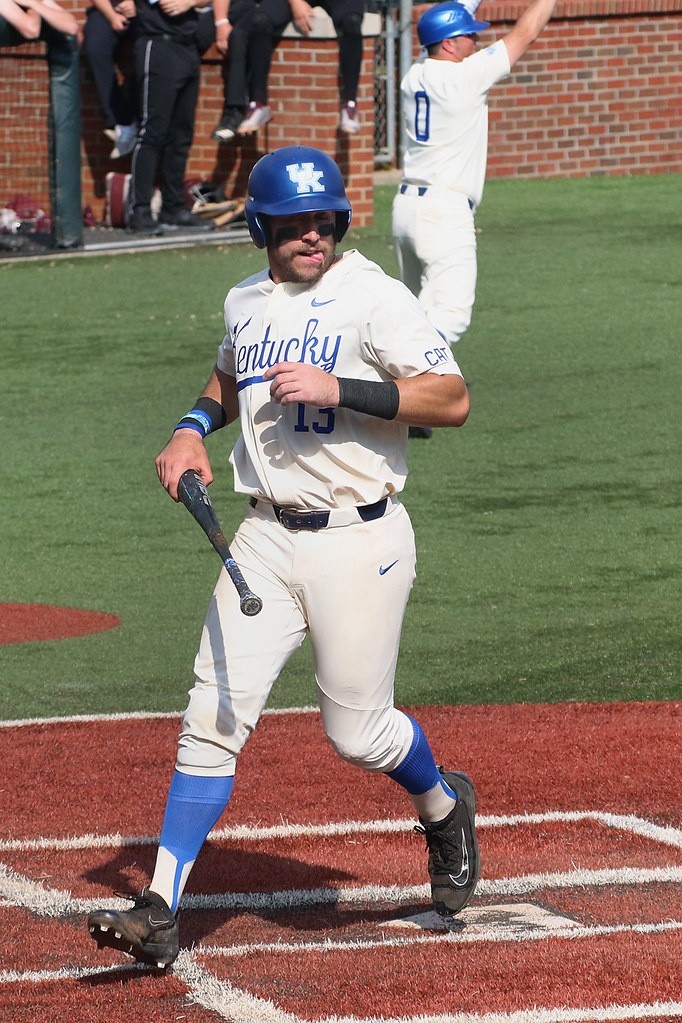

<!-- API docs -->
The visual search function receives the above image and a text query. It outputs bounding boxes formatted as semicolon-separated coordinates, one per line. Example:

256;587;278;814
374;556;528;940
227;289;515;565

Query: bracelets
175;410;212;438
215;18;229;26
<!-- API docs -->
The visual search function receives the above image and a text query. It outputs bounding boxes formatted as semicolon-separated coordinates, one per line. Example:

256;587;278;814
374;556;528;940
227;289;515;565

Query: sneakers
109;119;140;159
210;107;245;143
87;884;181;971
415;765;480;918
236;102;274;137
339;100;360;135
104;129;116;142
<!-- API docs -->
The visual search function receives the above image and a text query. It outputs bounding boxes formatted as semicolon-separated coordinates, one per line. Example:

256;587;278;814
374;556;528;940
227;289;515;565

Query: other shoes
158;209;214;230
127;212;162;235
409;426;432;438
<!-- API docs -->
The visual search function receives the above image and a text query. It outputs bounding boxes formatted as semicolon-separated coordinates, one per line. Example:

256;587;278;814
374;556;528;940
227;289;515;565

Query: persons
391;0;556;347
87;145;481;969
83;0;137;160
196;0;256;143
237;0;364;137
125;0;215;237
0;0;85;251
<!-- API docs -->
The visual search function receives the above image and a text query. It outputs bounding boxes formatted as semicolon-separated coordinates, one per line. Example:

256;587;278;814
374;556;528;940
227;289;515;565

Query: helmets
417;2;491;46
244;146;352;249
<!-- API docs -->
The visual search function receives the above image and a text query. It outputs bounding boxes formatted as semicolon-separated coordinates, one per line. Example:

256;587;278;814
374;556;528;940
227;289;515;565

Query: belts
154;33;190;47
249;496;388;532
400;185;473;210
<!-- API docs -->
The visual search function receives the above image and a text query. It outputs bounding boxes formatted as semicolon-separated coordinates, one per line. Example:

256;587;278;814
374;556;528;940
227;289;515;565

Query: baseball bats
177;468;263;618
